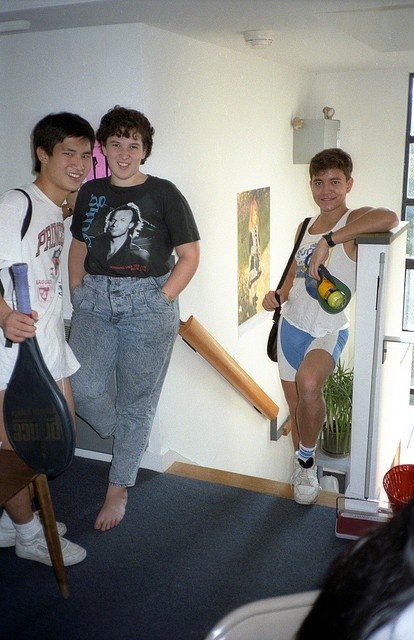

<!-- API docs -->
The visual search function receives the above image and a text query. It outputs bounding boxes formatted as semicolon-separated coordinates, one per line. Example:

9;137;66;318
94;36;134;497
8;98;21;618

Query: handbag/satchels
265;217;311;363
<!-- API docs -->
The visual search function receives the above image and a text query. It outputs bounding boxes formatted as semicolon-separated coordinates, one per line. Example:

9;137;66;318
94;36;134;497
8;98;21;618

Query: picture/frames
237;187;270;325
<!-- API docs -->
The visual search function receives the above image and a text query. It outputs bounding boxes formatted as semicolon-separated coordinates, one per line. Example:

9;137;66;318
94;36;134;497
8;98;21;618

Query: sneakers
15;529;87;568
293;458;320;506
1;509;68;548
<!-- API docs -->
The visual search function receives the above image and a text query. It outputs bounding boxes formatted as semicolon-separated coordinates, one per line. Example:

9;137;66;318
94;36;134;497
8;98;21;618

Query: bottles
316;271;347;310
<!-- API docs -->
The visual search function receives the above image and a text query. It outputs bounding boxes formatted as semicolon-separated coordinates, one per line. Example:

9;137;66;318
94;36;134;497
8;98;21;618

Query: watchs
324;229;336;248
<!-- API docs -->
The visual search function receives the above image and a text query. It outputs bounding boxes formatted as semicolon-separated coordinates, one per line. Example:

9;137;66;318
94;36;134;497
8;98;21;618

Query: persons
290;494;412;640
263;147;399;505
69;106;201;531
0;111;94;568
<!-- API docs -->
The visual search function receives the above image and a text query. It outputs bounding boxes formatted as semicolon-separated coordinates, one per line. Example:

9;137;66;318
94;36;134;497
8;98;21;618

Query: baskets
382;464;414;517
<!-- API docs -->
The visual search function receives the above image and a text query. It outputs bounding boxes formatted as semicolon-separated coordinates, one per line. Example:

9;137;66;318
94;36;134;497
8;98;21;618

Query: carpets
0;457;353;639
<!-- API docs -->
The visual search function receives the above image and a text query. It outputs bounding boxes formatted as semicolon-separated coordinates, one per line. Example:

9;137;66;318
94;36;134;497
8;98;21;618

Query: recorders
335;494;390;540
